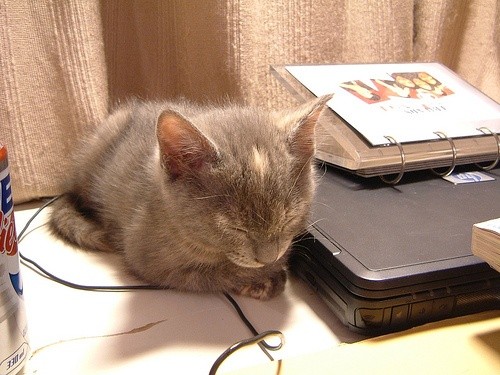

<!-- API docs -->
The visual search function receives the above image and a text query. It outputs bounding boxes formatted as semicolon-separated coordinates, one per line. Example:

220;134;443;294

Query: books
265;63;500;185
471;218;500;273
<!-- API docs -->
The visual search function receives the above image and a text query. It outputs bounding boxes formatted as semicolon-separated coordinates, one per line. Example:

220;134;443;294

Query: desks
10;194;500;375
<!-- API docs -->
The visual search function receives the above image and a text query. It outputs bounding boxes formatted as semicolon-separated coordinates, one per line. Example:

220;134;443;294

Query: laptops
287;160;500;335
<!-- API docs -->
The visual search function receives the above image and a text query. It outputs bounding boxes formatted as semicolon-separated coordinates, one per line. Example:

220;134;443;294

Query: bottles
0;142;32;375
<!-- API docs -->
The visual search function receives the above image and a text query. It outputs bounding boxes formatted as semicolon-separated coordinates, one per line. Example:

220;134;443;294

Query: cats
46;87;334;301
